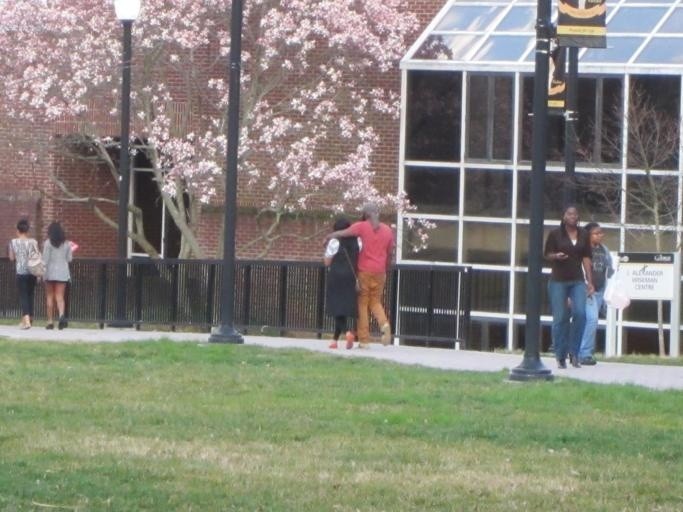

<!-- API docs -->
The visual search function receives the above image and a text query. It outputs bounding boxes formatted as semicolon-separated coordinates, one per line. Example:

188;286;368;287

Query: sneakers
328;324;391;350
17;316;69;330
555;356;596;368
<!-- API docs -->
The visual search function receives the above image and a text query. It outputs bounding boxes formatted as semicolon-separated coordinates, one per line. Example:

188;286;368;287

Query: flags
556;0;608;50
547;25;568;119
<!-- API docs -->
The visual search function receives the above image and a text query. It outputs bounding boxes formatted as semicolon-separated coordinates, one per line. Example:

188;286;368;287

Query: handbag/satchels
355;277;362;293
603;273;630;309
27;245;46;278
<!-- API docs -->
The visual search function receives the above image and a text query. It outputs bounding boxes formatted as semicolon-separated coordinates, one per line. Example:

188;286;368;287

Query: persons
38;220;73;331
540;203;595;370
577;221;614;367
320;216;363;351
319;204;396;352
7;218;40;331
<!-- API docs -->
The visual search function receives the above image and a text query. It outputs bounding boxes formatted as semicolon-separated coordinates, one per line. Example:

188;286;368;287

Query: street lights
111;1;141;327
